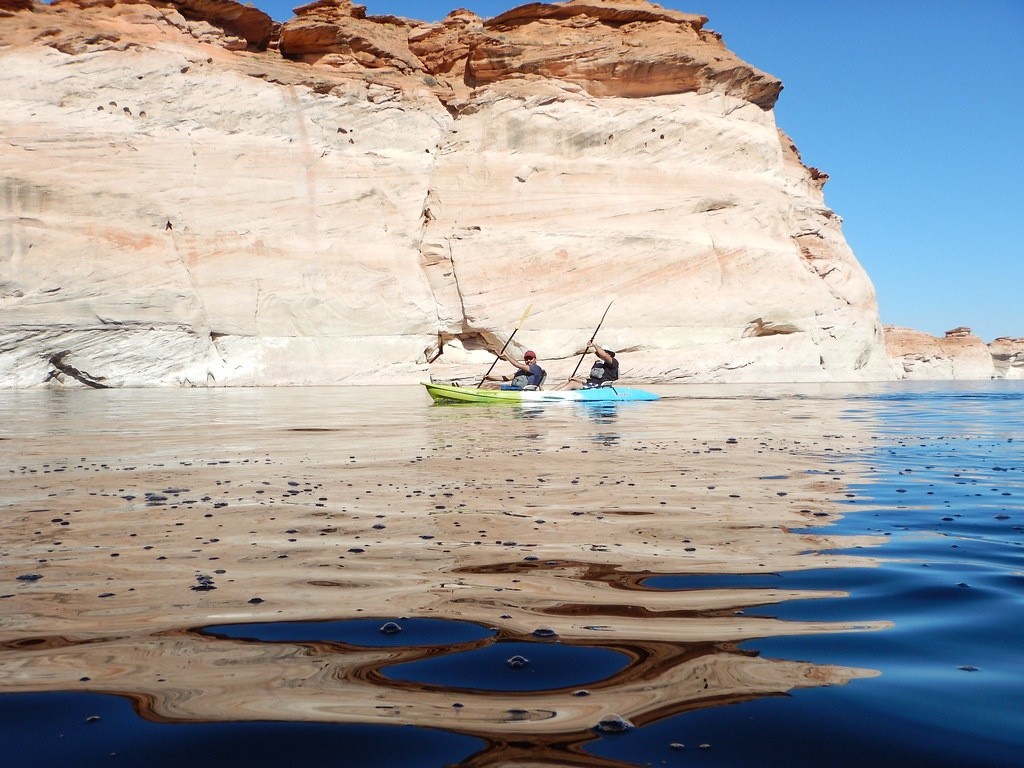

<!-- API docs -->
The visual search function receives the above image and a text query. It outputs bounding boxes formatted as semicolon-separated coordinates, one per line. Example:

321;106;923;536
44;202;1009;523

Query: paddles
476;304;533;390
550;300;614;390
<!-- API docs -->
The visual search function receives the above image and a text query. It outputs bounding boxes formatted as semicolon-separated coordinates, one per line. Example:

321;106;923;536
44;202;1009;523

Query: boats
420;381;664;405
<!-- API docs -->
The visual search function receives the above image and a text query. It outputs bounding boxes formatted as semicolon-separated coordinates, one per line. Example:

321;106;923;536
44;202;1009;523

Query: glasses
525;357;536;361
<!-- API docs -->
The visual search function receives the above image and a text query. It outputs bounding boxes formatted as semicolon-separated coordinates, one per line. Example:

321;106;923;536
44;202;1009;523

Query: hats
595;345;615;358
524;350;536;359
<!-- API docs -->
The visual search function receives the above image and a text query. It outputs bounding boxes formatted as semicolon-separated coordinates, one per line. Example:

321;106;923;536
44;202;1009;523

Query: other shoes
456;382;459;387
452;382;455;387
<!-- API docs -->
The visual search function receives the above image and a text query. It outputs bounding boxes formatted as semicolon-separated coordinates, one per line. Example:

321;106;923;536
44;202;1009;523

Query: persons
550;340;619;391
452;351;542;391
